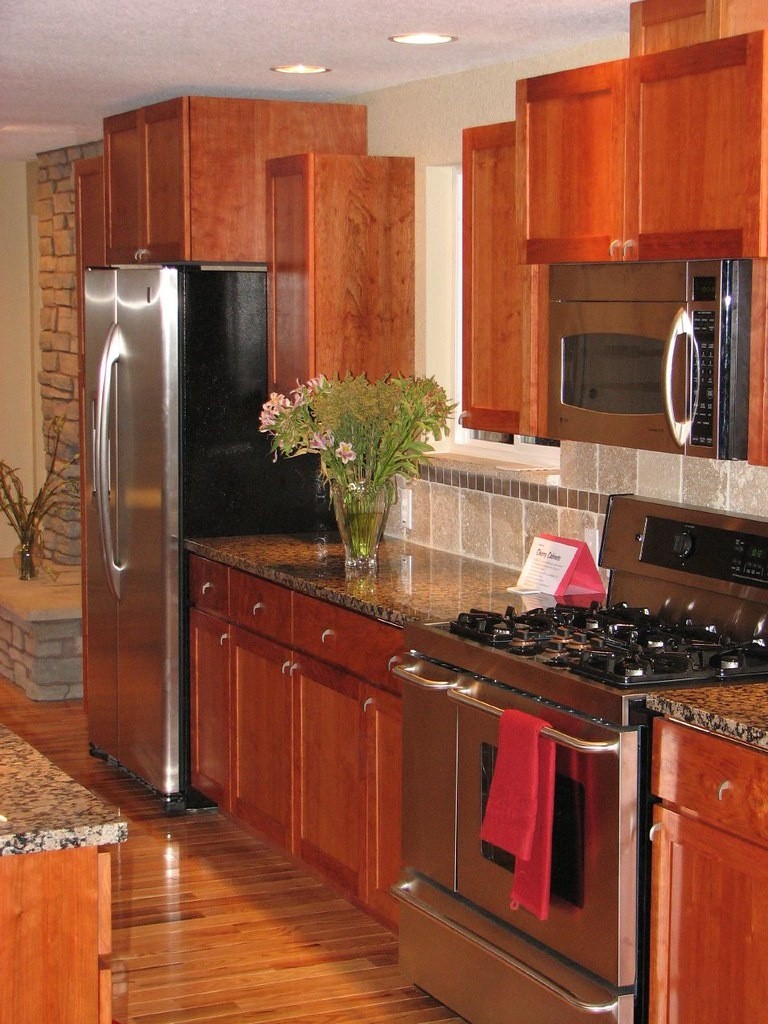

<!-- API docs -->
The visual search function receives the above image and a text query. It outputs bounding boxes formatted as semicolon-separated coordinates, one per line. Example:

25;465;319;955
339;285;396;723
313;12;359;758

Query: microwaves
545;256;754;462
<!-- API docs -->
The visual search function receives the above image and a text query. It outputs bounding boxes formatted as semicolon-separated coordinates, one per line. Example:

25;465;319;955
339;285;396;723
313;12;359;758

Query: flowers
259;372;458;561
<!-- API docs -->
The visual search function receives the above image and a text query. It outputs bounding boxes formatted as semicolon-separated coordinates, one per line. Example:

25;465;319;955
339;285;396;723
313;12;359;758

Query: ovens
386;655;651;1024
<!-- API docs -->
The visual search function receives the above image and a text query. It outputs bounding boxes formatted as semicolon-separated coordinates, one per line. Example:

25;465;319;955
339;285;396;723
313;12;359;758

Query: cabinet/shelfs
515;1;768;264
462;120;526;434
188;552;404;936
103;96;368;263
266;152;415;403
0;844;114;1024
649;714;768;1024
74;155;105;713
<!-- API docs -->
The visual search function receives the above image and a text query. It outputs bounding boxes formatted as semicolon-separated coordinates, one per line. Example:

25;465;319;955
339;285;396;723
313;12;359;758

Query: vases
329;481;394;569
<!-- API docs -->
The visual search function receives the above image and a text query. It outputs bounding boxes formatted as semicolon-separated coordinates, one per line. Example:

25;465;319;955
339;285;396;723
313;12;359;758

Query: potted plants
1;402;79;581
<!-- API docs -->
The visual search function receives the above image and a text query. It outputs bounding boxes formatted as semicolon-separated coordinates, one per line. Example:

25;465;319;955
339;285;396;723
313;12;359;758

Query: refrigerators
80;261;270;817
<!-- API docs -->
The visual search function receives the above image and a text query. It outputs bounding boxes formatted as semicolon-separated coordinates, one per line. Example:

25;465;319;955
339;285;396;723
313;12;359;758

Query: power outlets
399;554;411;596
401;489;412;528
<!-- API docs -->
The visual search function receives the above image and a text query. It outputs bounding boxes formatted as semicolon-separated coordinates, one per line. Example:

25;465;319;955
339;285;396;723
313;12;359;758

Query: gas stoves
401;598;768;691
